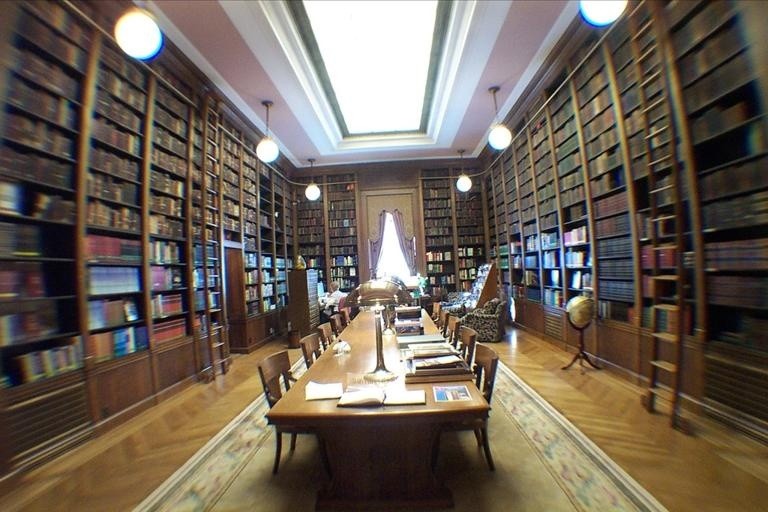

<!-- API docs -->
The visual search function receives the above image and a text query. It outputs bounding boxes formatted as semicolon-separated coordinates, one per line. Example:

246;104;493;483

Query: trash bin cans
289;330;301;348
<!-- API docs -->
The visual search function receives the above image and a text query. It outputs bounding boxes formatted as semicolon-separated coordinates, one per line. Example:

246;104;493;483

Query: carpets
126;329;671;512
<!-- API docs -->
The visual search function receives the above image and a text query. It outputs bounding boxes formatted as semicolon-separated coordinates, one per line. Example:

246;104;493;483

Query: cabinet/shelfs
288;269;323;339
469;262;498;310
566;1;699;401
478;43;596;365
80;2;226;435
294;158;486;299
647;0;768;436
219;91;296;365
2;1;104;497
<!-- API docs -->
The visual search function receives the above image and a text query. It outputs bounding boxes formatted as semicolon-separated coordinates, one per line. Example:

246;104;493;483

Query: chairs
255;302;499;511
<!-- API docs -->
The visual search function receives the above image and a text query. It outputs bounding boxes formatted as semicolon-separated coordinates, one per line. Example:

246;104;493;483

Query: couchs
437;291;508;343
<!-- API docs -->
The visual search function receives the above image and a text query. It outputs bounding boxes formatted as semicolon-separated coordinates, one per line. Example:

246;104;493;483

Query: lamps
487;85;513;150
305;157;320;200
456;148;472;193
255;100;279;164
346;279;415;382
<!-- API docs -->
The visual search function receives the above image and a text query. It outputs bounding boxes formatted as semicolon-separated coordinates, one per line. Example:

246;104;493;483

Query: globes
562;296;603;371
